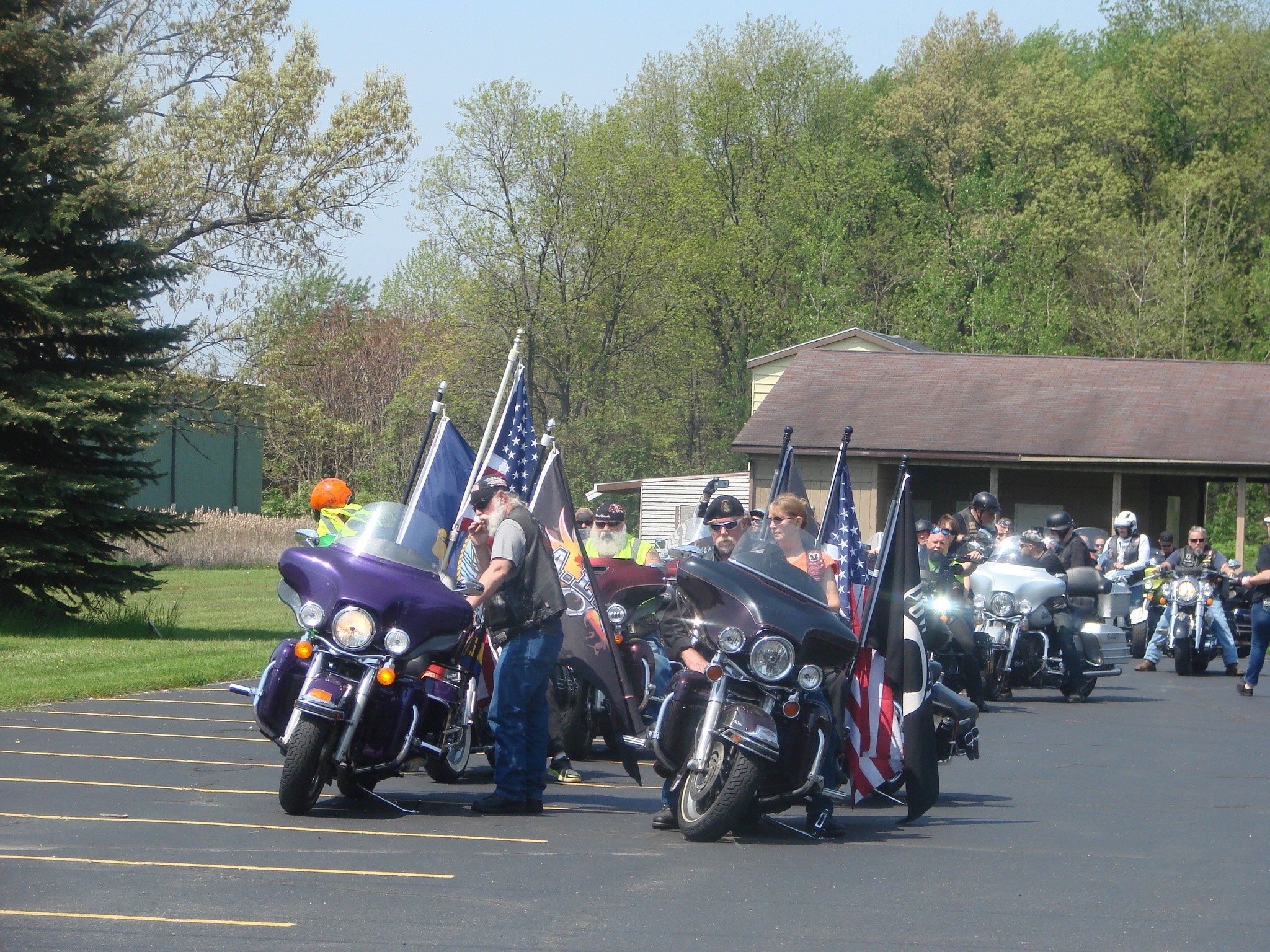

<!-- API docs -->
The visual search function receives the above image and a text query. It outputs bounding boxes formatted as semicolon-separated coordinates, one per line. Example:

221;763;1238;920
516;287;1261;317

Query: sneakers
547;755;582;783
652;803;679;828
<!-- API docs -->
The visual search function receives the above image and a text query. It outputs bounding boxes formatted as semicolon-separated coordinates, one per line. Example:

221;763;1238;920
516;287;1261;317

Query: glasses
472;494;494;512
1162;545;1172;548
576;521;594;526
996;533;1004;537
1018;544;1030;548
931;527;949;535
1189;538;1205;543
595;521;620;528
764;517;795;526
944;528;959;536
708;516;747;532
1093;544;1105;547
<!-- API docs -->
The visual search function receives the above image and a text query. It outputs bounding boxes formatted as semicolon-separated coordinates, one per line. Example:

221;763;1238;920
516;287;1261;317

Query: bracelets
1249;578;1254;586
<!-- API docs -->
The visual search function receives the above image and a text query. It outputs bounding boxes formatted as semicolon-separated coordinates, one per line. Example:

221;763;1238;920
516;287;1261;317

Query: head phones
1133;528;1140;538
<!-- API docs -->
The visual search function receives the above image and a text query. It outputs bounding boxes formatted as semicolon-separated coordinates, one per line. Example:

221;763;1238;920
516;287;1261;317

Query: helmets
1114;510;1137;535
750;508;766;520
915;519;934;531
310;478;356;512
972;492;1001;512
1045;511;1076;530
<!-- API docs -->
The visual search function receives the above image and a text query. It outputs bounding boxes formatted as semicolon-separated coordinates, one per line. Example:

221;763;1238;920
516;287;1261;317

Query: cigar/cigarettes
475;520;486;533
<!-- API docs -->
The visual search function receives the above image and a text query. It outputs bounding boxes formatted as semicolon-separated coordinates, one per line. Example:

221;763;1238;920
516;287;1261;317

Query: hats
595;502;625;521
574;508;594;521
997;517;1013;528
1159;531;1174;543
1021;529;1043;542
703;496;744;525
470;474;510;504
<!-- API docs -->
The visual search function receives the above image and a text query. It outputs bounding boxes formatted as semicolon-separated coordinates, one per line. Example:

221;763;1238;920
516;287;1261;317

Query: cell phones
714;480;729;488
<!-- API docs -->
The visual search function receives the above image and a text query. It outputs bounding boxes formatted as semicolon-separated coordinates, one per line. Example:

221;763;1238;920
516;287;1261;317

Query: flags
759;443;939;828
397;368;646;786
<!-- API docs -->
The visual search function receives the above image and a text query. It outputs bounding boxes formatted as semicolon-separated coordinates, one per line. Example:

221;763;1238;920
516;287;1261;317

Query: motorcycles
863;527;1256;806
547;515;716;759
230;500;496;816
622;520;851;843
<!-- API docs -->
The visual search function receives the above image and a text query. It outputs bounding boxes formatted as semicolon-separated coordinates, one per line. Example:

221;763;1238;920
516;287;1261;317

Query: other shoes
995;689;1013;701
807;812;845;836
968;698;989;712
1134;659;1156;671
1066;687;1082;703
1236;678;1253;696
471;792;544;814
1224;664;1246;676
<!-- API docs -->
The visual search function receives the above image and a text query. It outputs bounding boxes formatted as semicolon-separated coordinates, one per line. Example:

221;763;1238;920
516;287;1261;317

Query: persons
913;493;1106;702
574;501;674;738
547;689;581;782
1236;510;1270;696
651;479;849;837
1133;526;1246;676
1102;511;1150;646
461;474;580;816
1149;531;1177;565
